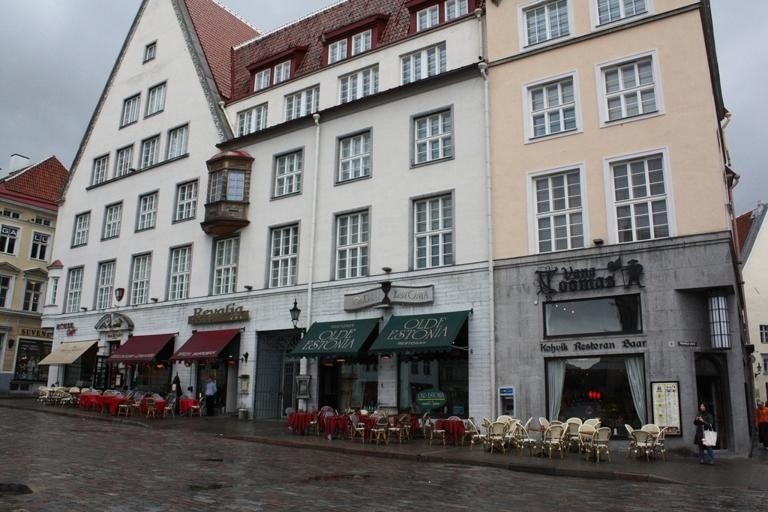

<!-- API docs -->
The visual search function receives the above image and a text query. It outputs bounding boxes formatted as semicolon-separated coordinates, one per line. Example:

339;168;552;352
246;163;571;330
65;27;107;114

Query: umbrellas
172;372;182;414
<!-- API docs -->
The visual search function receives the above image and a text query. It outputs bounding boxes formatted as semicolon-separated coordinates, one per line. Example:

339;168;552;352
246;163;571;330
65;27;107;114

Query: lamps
114;287;124;302
707;296;732;349
290;298;305;332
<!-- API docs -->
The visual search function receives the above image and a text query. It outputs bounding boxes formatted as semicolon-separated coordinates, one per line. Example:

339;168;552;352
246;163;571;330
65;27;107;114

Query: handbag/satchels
702;430;718;447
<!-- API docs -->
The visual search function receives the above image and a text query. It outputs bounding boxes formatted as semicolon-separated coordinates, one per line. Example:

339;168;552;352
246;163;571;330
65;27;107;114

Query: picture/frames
650;380;683;438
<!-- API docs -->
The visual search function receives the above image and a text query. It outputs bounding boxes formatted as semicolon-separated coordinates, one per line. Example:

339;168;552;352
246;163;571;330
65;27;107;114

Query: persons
753;400;768;450
182;387;199;416
203;375;218;416
692;401;715;466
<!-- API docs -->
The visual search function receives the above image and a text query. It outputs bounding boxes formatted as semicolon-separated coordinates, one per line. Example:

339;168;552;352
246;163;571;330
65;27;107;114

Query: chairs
36;385;206;419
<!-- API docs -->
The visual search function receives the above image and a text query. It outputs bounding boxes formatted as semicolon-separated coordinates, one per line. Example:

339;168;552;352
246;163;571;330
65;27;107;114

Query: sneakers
700;460;704;464
709;460;714;465
